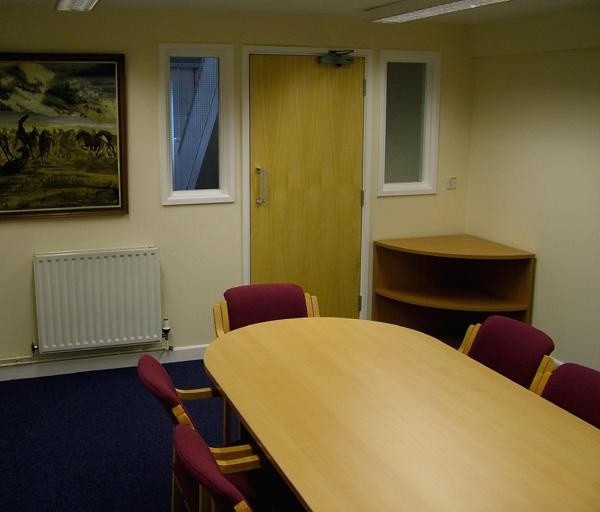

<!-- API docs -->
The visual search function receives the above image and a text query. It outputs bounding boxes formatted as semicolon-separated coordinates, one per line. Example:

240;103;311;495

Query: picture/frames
0;52;129;220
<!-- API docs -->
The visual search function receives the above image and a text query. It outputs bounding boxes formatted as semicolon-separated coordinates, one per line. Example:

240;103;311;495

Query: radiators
33;246;162;354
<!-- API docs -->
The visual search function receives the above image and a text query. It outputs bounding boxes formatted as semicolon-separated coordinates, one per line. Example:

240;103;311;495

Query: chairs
212;282;321;446
138;353;257;512
535;358;600;429
458;313;555;391
173;423;270;512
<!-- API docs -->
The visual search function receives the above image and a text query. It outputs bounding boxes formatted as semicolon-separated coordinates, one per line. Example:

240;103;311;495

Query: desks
203;315;600;512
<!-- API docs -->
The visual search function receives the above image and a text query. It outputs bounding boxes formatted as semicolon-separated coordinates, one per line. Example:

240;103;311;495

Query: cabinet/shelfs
370;236;536;349
